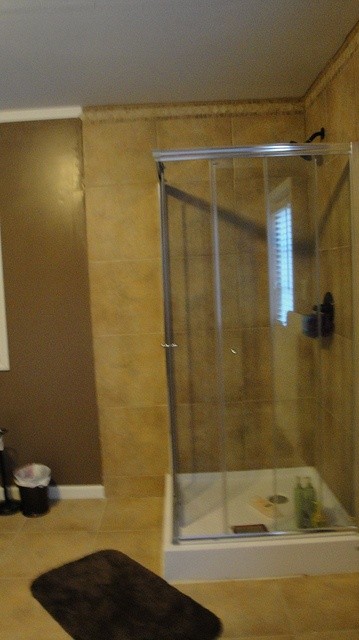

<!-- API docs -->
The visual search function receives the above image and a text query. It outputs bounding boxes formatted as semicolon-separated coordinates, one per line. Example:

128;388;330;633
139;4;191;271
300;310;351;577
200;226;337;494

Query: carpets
30;550;222;640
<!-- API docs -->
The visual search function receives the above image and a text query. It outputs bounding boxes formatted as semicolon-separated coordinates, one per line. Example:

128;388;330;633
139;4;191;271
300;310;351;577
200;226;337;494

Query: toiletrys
294;475;306;529
306;475;318;528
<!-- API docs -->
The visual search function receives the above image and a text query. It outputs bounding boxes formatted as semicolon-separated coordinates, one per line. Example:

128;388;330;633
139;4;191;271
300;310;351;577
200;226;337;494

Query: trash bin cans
13;464;52;518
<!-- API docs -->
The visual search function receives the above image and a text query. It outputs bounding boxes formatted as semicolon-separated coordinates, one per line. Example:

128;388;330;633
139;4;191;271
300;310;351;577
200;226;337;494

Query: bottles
306;477;317;531
1;427;18;518
293;478;304;531
